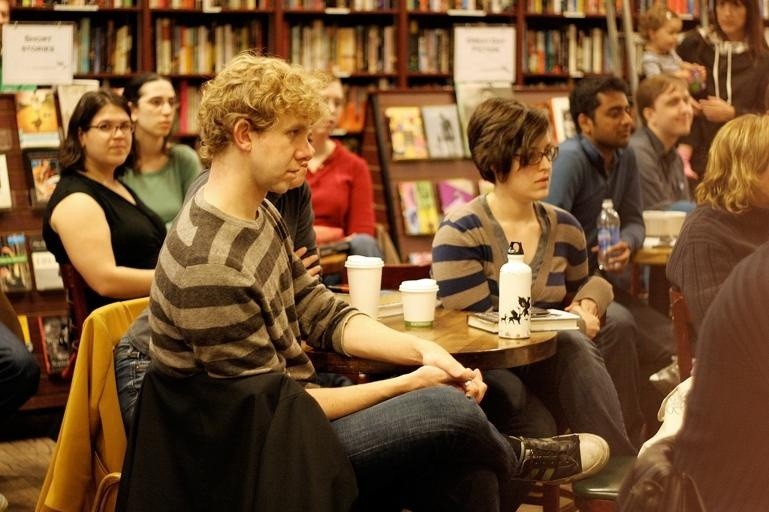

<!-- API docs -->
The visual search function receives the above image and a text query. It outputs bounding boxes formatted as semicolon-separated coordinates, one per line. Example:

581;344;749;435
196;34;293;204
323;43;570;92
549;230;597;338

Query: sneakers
649;356;696;397
508;432;610;484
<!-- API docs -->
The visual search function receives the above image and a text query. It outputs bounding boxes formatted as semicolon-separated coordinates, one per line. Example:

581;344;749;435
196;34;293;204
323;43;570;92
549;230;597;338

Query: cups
399;278;440;329
344;254;385;320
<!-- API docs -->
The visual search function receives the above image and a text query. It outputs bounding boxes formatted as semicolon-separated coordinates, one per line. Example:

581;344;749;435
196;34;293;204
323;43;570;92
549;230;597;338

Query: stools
574;453;637;512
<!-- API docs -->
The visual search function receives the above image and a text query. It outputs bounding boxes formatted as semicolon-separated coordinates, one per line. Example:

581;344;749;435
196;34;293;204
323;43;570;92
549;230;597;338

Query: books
523;0;715;77
385;103;494;266
0;0;142;377
551;97;576;143
466;306;582;334
149;0;516;137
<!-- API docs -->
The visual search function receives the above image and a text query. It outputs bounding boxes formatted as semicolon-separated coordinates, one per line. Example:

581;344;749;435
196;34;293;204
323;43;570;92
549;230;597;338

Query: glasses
515;146;558;165
90;122;134;133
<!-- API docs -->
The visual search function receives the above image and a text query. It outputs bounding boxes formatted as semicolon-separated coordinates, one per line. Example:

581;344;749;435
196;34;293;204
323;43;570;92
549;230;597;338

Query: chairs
33;268;573;512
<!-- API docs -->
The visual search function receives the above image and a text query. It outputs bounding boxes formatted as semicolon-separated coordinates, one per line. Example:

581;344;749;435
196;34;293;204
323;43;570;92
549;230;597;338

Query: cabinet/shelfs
0;0;769;412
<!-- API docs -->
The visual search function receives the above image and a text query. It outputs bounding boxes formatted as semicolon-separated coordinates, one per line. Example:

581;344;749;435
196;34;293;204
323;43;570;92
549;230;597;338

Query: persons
0;321;40;423
431;96;638;457
670;239;769;512
121;71;205;228
41;89;168;316
147;54;611;512
292;70;375;255
536;73;674;436
114;132;323;434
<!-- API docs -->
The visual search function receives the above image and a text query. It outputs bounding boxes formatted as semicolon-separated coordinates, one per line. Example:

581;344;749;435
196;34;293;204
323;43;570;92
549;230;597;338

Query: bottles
497;241;533;340
597;198;620;270
